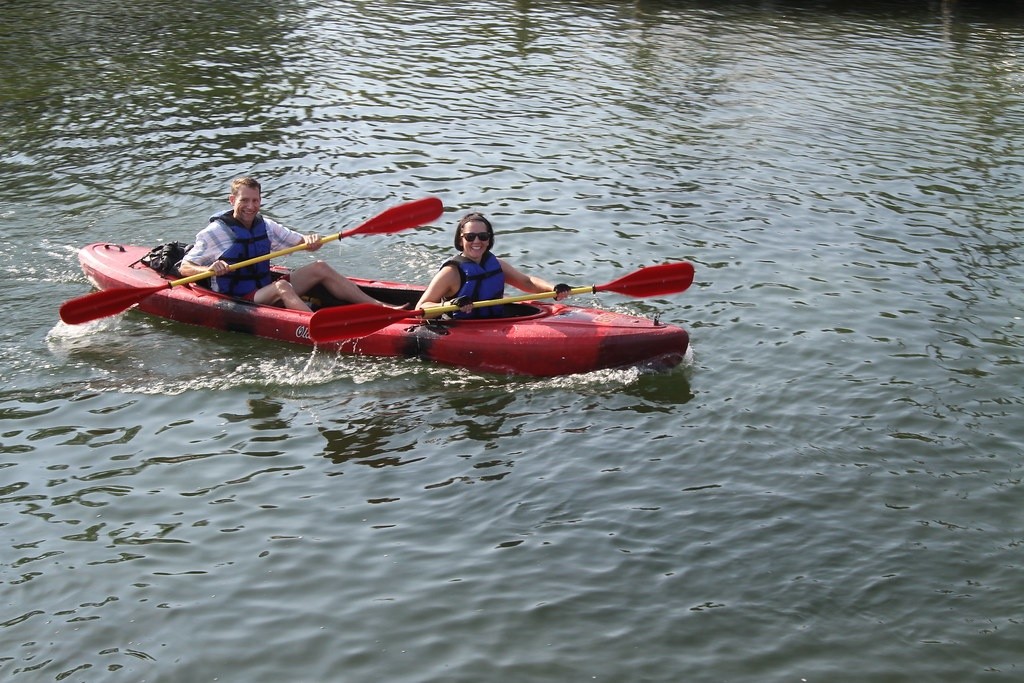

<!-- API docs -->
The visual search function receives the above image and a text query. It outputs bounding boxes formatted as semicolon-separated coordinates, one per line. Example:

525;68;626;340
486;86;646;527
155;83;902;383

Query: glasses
460;232;491;243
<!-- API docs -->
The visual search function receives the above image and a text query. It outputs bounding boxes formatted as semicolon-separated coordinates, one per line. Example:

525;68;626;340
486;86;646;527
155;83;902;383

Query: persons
414;212;572;319
178;177;411;311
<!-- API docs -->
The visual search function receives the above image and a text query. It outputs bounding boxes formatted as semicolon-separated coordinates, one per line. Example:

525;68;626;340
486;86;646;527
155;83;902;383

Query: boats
77;239;689;381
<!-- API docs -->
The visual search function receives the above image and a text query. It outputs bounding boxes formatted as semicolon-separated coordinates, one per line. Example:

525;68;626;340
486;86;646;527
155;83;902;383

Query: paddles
307;263;694;348
58;196;446;327
